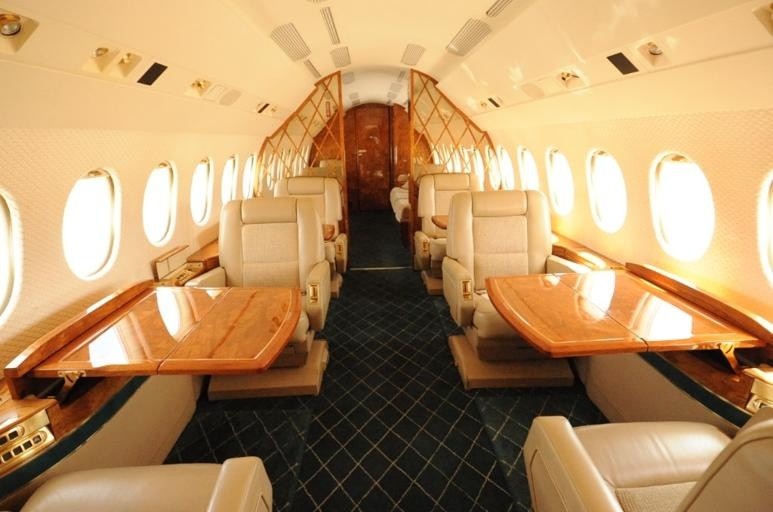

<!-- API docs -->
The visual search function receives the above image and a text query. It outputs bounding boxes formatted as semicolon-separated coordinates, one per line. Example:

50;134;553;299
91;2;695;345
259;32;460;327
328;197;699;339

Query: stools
19;457;273;512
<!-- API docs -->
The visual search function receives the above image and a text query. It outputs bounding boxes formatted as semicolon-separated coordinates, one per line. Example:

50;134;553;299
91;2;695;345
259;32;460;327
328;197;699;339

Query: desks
485;267;767;375
26;285;302;403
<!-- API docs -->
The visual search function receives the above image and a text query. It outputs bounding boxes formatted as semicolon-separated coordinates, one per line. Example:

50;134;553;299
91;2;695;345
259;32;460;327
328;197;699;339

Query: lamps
648;42;663;55
0;13;21;36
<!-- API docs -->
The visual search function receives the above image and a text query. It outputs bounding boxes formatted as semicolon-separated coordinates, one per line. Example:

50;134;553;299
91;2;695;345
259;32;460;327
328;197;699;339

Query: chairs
522;406;773;512
389;163;447;235
184;159;347;402
412;172;479;289
441;188;591;361
